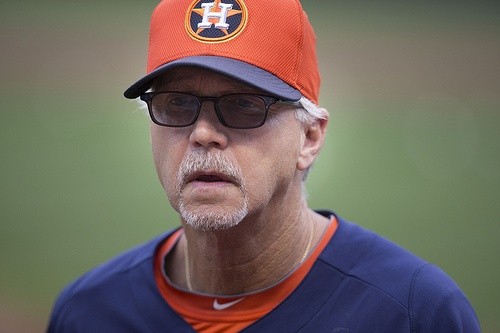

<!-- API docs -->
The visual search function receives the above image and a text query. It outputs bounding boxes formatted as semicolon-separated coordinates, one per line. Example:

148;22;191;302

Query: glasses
139;90;280;129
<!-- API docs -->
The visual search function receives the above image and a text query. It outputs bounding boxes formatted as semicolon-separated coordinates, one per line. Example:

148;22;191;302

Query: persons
46;0;482;332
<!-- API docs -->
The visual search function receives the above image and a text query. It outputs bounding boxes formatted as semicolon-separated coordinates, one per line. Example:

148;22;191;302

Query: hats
123;0;321;106
